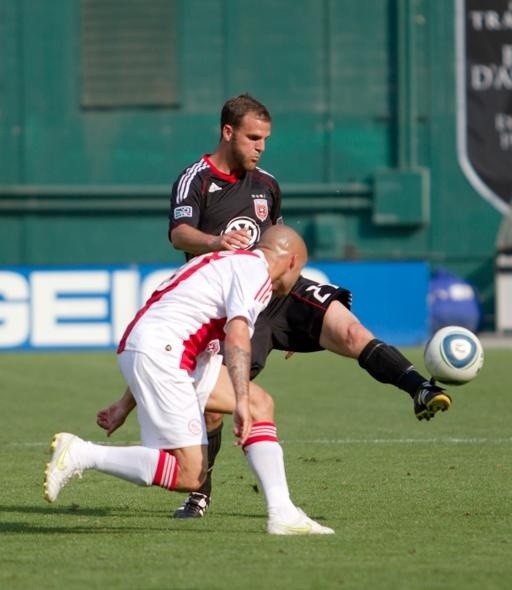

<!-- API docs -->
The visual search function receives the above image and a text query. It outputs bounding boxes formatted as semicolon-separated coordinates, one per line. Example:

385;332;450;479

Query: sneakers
265;509;334;537
43;432;81;502
173;491;211;519
414;380;452;422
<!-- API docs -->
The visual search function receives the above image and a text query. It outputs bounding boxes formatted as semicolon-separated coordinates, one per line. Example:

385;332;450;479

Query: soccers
423;325;484;386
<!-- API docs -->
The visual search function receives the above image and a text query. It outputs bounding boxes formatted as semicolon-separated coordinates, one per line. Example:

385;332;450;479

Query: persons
42;224;336;537
168;93;453;521
